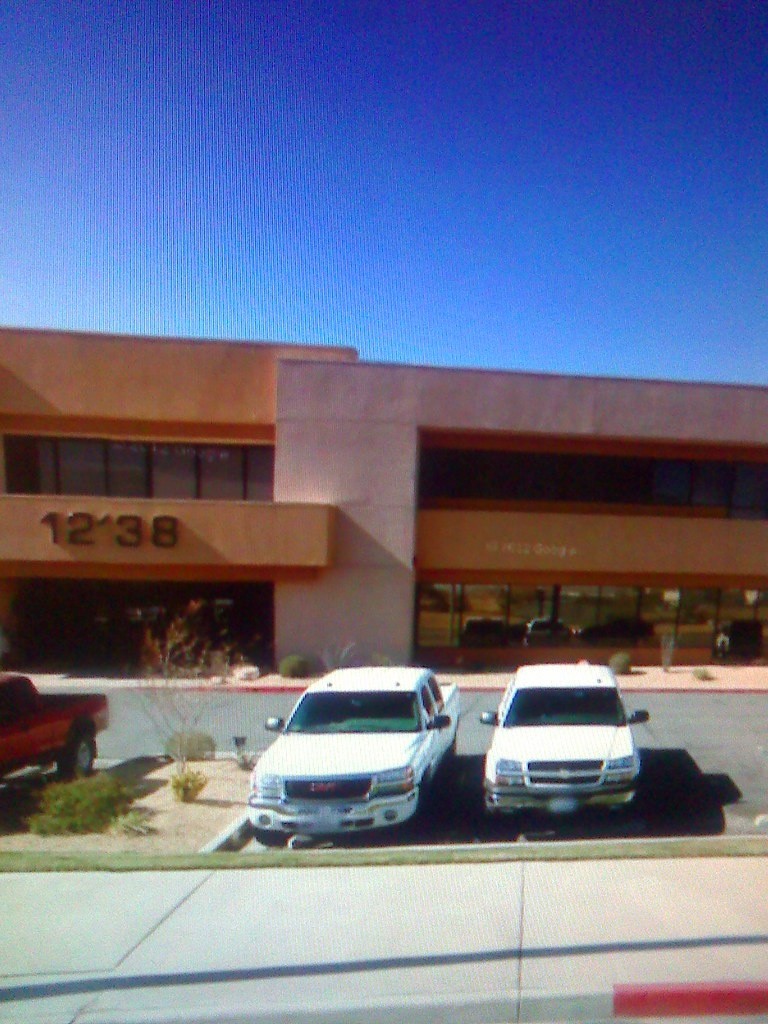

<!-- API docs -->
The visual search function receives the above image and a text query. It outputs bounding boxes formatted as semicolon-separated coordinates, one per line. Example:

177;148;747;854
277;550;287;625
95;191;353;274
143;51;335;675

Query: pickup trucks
0;673;108;783
245;664;462;845
481;661;651;815
